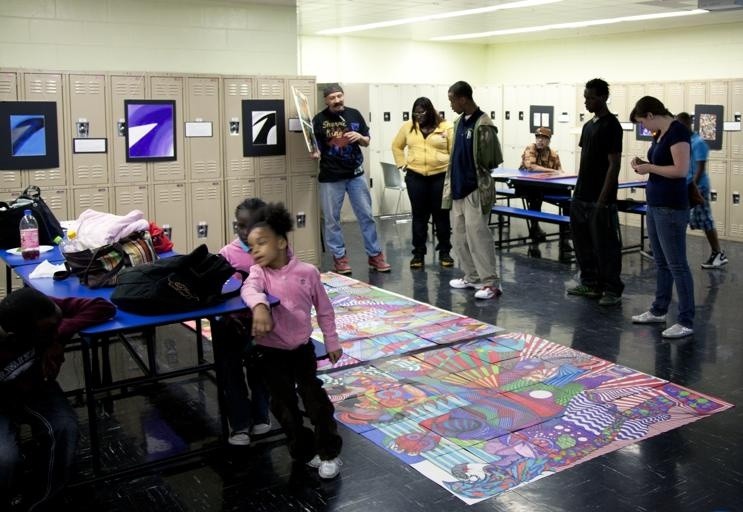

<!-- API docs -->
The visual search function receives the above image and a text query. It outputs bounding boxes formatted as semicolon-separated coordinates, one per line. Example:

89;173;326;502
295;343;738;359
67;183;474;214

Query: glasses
413;110;428;117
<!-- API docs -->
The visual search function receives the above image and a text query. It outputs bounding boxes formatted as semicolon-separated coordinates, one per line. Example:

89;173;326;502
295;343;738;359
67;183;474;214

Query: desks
0;223;67;302
6;249;296;500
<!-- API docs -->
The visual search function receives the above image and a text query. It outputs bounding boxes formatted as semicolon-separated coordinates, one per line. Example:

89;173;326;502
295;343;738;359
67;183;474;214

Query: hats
322;83;343;97
535;127;551;138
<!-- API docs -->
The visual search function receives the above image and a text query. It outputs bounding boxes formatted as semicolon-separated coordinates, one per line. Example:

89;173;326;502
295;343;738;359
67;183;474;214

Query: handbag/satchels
0;185;66;252
52;208;174;288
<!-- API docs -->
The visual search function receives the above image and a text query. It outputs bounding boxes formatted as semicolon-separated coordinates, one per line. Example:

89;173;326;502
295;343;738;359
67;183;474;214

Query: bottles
52;236;80;258
64;229;94;255
18;208;39;261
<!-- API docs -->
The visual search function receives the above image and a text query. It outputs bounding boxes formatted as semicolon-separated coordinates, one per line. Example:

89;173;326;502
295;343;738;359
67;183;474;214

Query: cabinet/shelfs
315;76;742;245
0;67;321;338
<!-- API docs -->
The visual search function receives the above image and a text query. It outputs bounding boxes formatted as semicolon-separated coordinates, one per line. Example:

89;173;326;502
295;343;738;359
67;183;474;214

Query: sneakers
473;282;503;300
566;284;600;297
640;248;656;261
333;249;354;275
631;310;667;324
409;255;424;268
439;255;455;267
661;322;694;338
227;427;250;446
449;277;483;289
599;291;622;306
252;414;273;436
530;227;546;239
306;453;322;468
318;453;343;479
367;252;393;272
701;249;729;268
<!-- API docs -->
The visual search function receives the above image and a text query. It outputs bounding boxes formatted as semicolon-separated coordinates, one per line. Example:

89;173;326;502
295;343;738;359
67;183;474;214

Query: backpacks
108;244;248;312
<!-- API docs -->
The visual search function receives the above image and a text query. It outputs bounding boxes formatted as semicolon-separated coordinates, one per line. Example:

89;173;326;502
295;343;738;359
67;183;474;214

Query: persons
0;288;117;512
241;201;343;479
567;78;626;305
213;198;293;445
640;112;728;268
441;81;504;299
391;96;455;266
629;96;695;339
311;84;391;274
514;127;574;252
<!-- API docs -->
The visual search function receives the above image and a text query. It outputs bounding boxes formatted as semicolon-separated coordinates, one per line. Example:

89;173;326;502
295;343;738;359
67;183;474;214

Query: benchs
308;335;331;361
427;163;657;277
